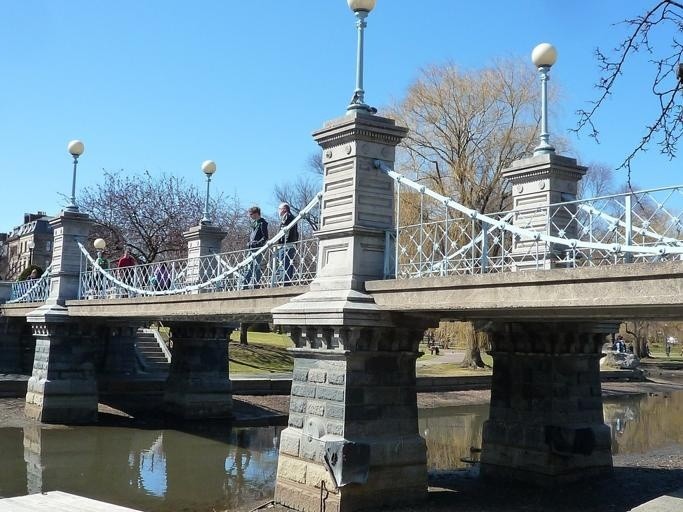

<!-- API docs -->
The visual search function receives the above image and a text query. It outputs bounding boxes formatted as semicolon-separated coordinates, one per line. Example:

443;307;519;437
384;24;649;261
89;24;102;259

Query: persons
93;251;108;290
428;347;433;355
23;269;38;302
118;251;134;297
277;203;297;286
435;347;438;354
242;207;267;290
616;335;683;359
149;261;169;291
615;416;626;436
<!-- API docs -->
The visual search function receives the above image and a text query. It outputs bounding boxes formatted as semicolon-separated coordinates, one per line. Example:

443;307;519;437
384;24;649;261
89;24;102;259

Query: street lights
64;140;84;213
27;241;35;266
664;323;667;355
198;159;215;227
345;0;379;118
271;425;277;450
423;408;430;436
531;43;557;158
91;238;106;277
